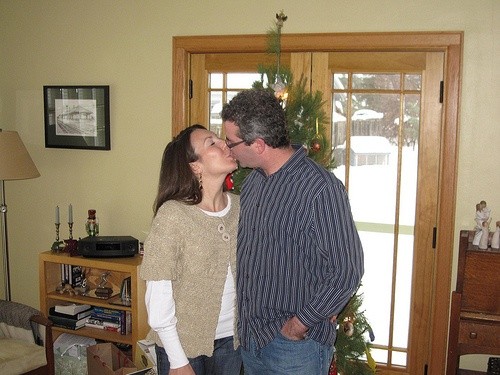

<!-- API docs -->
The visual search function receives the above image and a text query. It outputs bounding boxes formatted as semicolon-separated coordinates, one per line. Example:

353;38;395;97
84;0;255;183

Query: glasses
225;135;249;149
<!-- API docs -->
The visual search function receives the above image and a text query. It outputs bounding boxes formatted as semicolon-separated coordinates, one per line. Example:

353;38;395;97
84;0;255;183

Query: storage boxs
86;339;156;375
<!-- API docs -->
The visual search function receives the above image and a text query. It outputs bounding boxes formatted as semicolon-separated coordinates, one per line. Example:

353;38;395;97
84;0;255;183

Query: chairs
0;299;55;375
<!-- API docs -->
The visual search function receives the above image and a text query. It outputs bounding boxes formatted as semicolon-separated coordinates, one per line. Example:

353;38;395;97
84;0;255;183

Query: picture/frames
43;85;110;150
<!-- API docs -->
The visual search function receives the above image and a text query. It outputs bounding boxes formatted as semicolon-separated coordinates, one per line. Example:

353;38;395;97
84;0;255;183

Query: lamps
0;128;41;302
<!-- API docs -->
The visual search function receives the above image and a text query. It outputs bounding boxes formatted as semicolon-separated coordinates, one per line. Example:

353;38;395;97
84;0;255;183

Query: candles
68;203;72;223
87;209;96;219
55;205;60;224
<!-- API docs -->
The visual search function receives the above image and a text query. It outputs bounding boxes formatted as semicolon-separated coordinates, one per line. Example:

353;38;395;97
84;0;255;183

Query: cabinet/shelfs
39;250;152;370
447;230;500;375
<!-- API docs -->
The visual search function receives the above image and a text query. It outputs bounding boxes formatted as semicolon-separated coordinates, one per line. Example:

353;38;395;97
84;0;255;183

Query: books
47;302;132;337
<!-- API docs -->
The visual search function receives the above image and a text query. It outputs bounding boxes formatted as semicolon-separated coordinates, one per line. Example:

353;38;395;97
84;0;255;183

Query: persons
222;90;365;375
140;124;243;375
472;200;500;250
